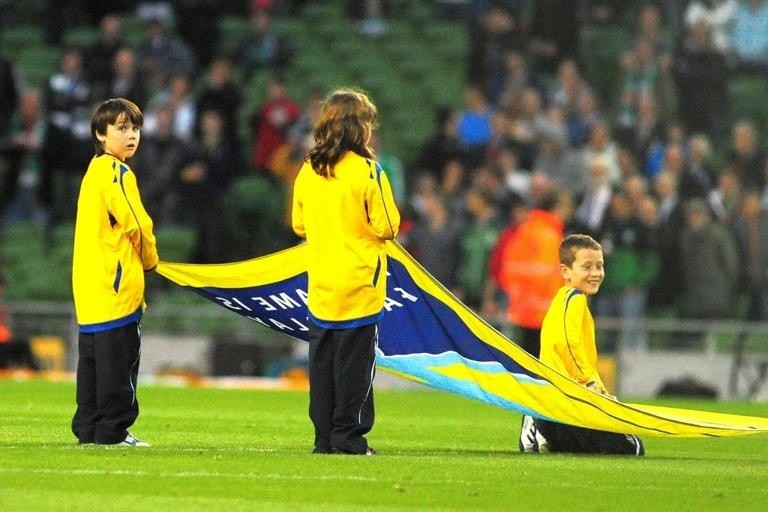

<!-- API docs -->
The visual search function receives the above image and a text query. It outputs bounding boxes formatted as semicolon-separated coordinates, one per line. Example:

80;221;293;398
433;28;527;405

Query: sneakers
365;445;376;456
520;414;539;453
542;441;556;452
116;433;149;447
